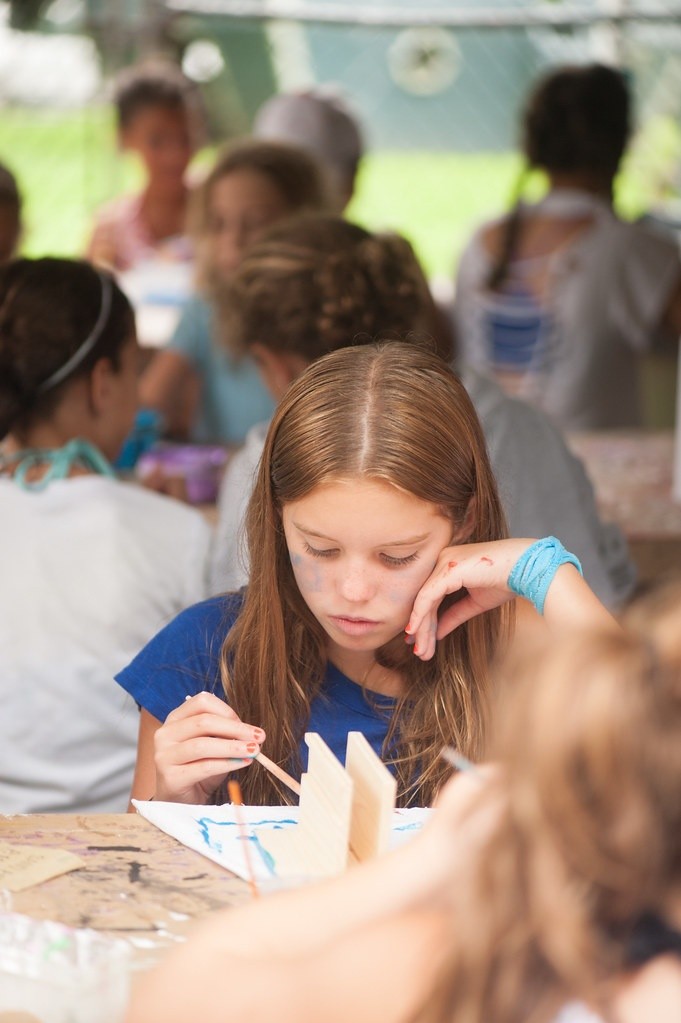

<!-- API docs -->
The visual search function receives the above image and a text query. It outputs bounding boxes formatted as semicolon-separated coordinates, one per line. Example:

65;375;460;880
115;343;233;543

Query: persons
464;60;681;433
0;76;640;811
136;576;681;1022
115;342;625;814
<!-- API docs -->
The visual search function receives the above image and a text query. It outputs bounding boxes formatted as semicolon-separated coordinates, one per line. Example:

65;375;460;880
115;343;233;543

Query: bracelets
502;537;587;611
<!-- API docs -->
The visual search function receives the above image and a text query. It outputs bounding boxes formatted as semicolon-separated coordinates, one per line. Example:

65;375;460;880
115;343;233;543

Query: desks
0;807;432;1022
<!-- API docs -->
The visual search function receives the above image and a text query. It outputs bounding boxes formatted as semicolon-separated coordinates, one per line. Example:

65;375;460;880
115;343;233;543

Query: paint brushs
185;697;300;796
226;781;257;901
437;747;474;771
